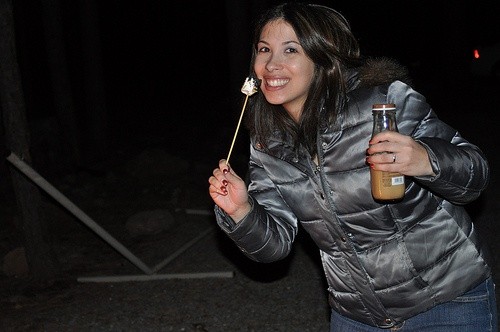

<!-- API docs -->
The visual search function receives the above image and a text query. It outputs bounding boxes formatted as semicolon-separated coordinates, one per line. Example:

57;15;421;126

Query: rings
392;152;396;163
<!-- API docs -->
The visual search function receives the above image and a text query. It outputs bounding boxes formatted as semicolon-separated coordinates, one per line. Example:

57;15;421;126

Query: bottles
369;104;405;203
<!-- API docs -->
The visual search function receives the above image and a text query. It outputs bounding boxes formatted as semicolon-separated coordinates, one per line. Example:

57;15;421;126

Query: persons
207;3;499;332
